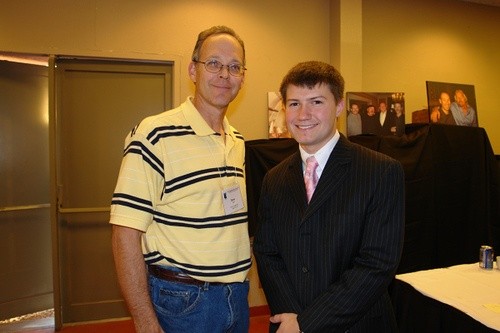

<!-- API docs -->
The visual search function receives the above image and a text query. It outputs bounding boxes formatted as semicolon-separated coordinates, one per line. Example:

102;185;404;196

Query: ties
304;156;318;204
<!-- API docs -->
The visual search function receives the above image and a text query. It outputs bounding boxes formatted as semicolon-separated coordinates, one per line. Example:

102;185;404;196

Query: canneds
479;245;494;270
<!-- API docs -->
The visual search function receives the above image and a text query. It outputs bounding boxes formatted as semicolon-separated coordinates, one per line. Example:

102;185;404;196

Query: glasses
194;60;247;76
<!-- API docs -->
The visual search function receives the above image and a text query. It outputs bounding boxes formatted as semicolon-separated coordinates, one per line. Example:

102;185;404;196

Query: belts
147;265;222;286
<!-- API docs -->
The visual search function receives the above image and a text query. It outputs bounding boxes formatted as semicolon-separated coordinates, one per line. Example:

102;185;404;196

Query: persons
253;60;405;333
430;91;455;125
109;25;254;333
362;101;405;135
348;104;362;136
433;89;476;126
